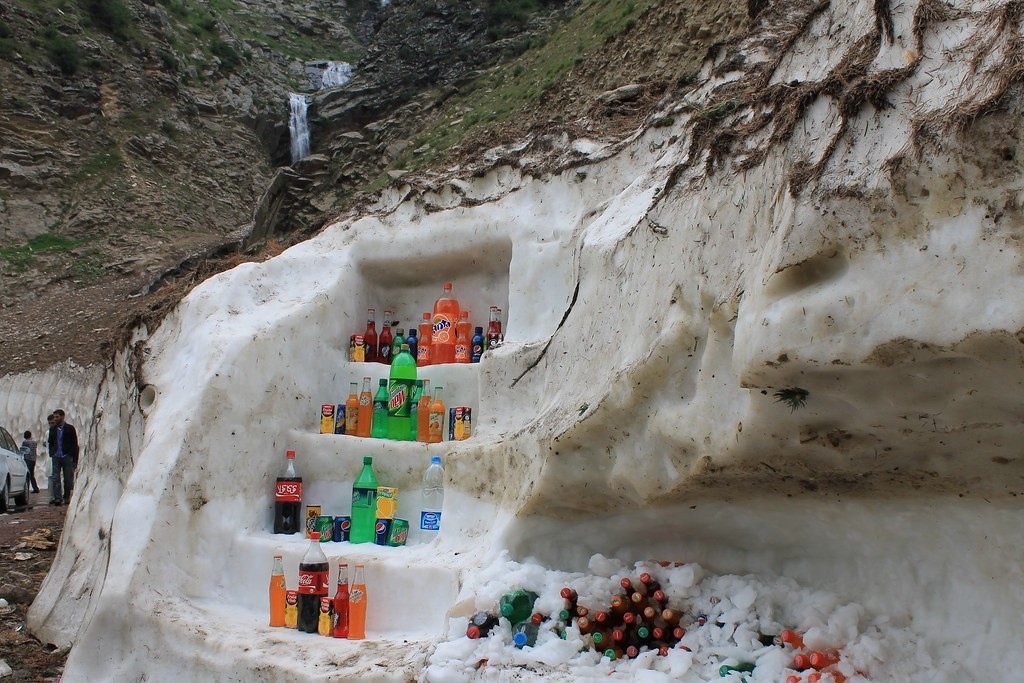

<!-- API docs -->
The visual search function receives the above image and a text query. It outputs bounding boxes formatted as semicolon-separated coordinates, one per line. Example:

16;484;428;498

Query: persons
21;431;40;493
44;409;79;506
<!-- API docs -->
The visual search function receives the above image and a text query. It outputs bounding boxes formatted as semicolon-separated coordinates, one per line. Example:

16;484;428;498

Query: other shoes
31;490;40;493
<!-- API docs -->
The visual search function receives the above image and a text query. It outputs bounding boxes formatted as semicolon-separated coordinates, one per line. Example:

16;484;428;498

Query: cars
0;425;32;513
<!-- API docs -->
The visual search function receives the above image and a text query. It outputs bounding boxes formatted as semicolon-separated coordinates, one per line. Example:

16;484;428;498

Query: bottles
297;532;330;633
429;386;446;444
419;455;445;546
273;451;303;534
334;563;348;639
466;574;863;682
348;565;367;639
357;377;372;436
269;555;286;627
370;379;389;439
387;344;417;440
410;379;423;442
365;283;501;368
417;379;433;442
345;382;359;436
350;456;377;545
